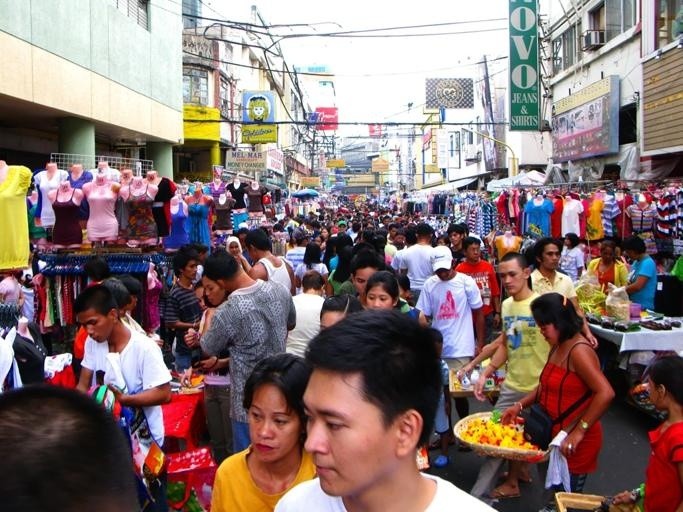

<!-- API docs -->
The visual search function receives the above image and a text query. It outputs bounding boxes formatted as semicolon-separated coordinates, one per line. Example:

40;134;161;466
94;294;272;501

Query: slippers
432;453;451;467
500;471;534;485
484;487;521;501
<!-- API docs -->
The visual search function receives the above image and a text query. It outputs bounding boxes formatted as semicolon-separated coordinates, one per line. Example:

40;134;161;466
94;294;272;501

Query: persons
493;182;683;257
1;161;267;271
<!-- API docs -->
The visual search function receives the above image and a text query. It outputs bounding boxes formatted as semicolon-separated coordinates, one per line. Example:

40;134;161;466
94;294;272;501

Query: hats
338;220;347;227
429;245;452;273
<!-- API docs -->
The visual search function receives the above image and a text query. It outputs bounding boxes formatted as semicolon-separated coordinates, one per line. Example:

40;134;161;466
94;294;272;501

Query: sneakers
538;501;558;512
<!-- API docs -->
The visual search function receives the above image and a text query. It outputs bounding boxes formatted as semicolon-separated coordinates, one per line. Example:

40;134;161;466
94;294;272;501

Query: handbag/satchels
523;402;553;452
85;382;173;507
168;296;193;356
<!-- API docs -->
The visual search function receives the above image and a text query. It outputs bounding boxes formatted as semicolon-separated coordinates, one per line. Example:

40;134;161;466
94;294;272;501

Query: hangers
40;252;168;275
0;272;34;339
500;180;682;198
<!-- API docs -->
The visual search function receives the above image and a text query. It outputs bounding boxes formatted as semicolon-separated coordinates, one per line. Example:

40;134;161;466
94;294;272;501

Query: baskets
452;411;551;464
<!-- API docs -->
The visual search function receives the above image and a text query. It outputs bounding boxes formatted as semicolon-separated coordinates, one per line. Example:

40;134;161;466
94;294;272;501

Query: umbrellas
292;190;319;198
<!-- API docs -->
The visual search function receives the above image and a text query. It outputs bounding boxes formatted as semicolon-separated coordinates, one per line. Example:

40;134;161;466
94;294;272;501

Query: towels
541;429;575;492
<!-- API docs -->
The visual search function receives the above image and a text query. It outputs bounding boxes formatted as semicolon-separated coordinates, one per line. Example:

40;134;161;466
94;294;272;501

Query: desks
579;297;682;406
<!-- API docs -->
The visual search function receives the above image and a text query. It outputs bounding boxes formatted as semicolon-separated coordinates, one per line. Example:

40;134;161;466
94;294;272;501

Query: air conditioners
579;30;607;51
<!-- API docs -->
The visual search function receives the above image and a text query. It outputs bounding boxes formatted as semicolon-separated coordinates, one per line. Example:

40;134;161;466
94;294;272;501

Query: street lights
458;125;517;176
